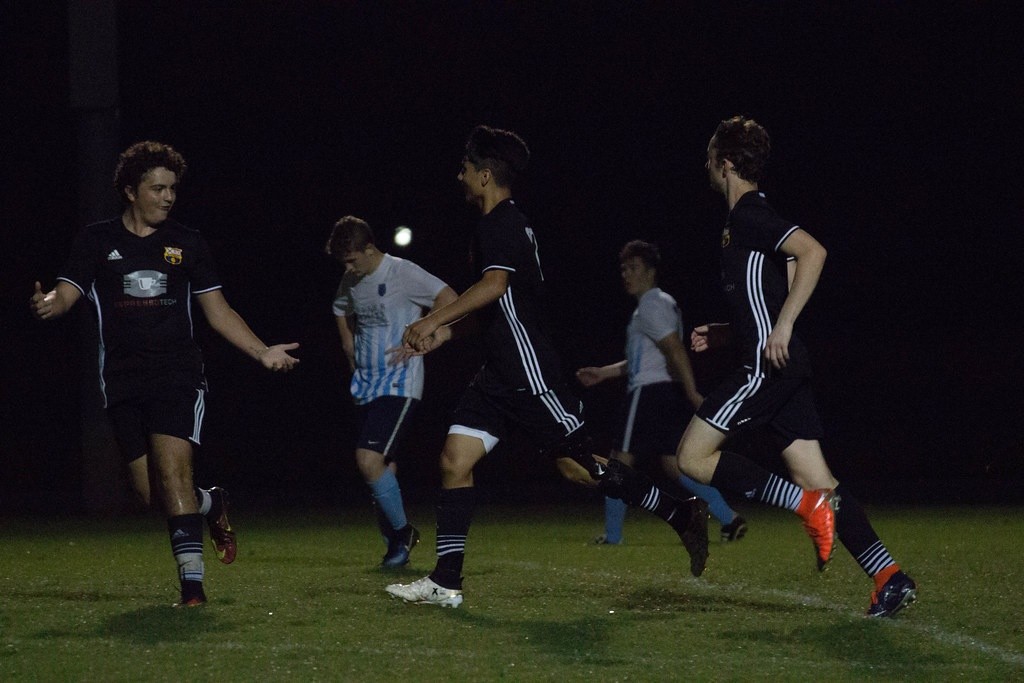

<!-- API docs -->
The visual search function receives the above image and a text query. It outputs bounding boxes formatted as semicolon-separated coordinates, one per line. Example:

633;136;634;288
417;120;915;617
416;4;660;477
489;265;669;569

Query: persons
385;125;712;609
578;237;751;549
27;140;304;610
327;214;469;568
674;115;921;620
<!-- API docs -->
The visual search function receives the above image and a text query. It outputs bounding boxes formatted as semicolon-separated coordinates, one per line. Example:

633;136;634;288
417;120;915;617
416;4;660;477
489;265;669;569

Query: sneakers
380;523;420;572
867;570;918;620
800;488;840;574
679;497;715;576
385;573;465;610
172;588;208;609
208;486;237;564
721;514;747;542
